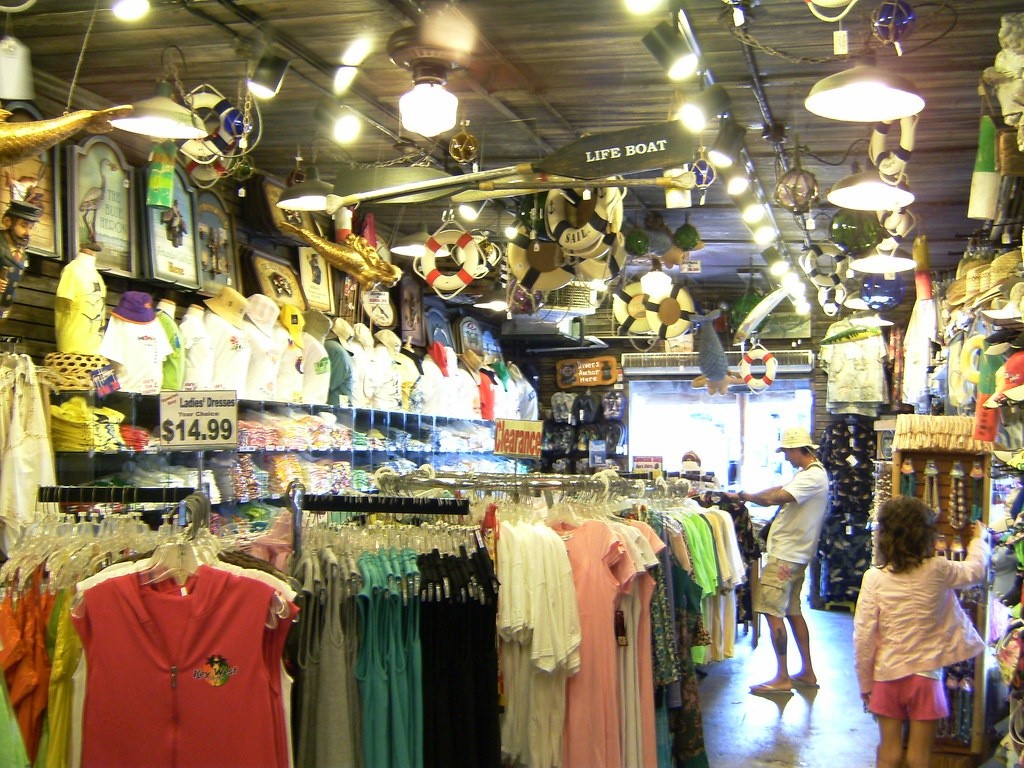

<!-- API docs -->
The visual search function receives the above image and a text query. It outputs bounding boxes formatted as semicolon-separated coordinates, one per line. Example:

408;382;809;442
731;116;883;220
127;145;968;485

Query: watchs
738;490;744;499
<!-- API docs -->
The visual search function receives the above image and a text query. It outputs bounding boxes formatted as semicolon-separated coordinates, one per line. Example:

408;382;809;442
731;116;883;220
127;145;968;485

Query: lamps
808;48;925;122
391;221;453;258
708;113;747;167
449;120;479;165
844;288;893;310
678;66;730;132
826;157;915;212
848;249;917;274
247;57;291;99
847;311;895;326
458;200;489;222
641;0;700;80
714;149;750;196
741;219;775;244
386;27;472;138
276;166;334;212
110;82;208;138
732;176;764;223
640;260;672;298
760;236;806;305
314;102;360;144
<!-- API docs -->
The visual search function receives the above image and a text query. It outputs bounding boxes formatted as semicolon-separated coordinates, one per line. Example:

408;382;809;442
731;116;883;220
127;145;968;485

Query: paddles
326;119;696;215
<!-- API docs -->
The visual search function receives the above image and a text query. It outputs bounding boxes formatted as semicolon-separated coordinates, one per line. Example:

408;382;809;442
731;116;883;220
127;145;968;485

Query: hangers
498;469;691;537
1;486;498;629
1;334;60;395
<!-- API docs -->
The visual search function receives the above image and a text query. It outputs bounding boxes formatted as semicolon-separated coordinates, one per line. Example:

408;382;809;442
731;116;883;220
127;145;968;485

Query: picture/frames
65;135;137;278
243;248;310;315
196;185;244;301
397;273;426;347
424;307;456;351
293;246;336;315
137;157;205;291
0;101;63;261
459;316;484;355
253;174;321;248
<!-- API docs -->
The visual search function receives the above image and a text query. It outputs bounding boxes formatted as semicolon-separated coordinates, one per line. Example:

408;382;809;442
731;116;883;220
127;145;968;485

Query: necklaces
936;658;974;744
901;473;983;529
934;534;964;562
959;586;980;627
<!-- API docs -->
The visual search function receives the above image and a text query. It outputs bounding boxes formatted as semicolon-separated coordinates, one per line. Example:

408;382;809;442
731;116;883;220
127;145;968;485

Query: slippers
601;389;626;422
576;424;601;452
550;391;579;426
543;422;576;455
572;392;603;424
550;458;620;476
750;684;792;693
789;675;820;689
603;422;627;455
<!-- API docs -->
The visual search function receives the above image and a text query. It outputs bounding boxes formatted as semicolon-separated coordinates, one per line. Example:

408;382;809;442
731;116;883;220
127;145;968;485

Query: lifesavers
960;334;986;384
508;176;627;291
739;348;777;391
804;242;850;289
334;207;378;250
613;281;695;339
457;235;498;276
170;91;238;181
421;229;478;292
869;115;917;175
876;181;909;250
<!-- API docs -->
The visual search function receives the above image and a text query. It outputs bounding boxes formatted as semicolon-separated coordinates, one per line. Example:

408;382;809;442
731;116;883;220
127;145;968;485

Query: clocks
359;285;397;330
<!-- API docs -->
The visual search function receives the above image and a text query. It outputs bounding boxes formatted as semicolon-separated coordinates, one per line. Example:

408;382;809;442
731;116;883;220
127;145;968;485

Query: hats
279;302;306;349
490;361;509;392
991;549;1017;598
202;286;251;330
302;308;333;345
993;697;1024;768
374;329;402;366
479;368;498;385
330;317;355;356
983;448;1024;605
400;346;424;375
427;341;450;377
458;349;482;386
244;293;280;339
993;619;1023;695
352;322;375;362
981;351;1024;409
930;228;1023;356
775;426;820;454
108;290;156;324
508;363;522;382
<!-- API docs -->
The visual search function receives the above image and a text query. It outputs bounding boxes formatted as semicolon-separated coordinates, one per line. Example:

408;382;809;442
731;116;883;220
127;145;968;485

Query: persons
724;426;829;693
99;286;538;421
852;496;989;768
54;247;107;355
0;199;43;324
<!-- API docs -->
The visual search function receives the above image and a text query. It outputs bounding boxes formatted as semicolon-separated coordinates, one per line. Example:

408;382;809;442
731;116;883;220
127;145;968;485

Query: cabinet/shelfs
50;386;532;498
